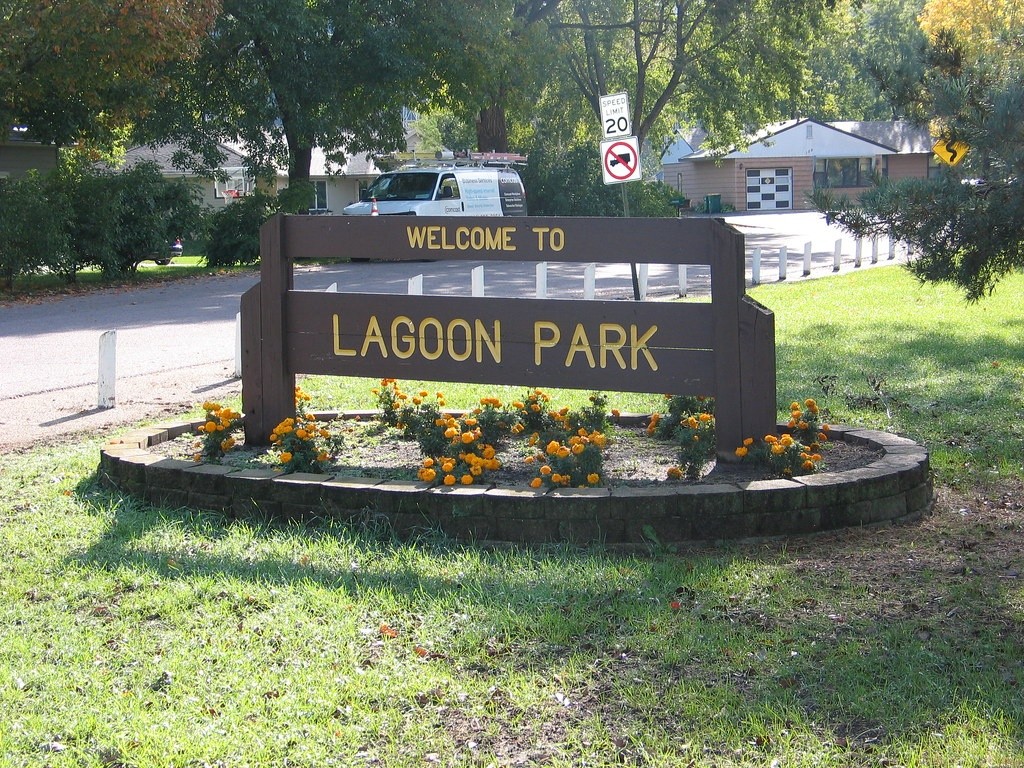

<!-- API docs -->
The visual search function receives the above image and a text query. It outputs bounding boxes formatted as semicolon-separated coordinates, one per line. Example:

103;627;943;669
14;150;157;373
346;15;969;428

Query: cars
144;236;183;265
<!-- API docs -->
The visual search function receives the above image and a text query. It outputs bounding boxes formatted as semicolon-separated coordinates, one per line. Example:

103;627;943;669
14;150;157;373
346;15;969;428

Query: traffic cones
367;197;379;216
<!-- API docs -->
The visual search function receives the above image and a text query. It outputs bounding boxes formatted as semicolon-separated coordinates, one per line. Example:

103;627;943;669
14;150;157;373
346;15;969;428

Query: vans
340;147;532;261
